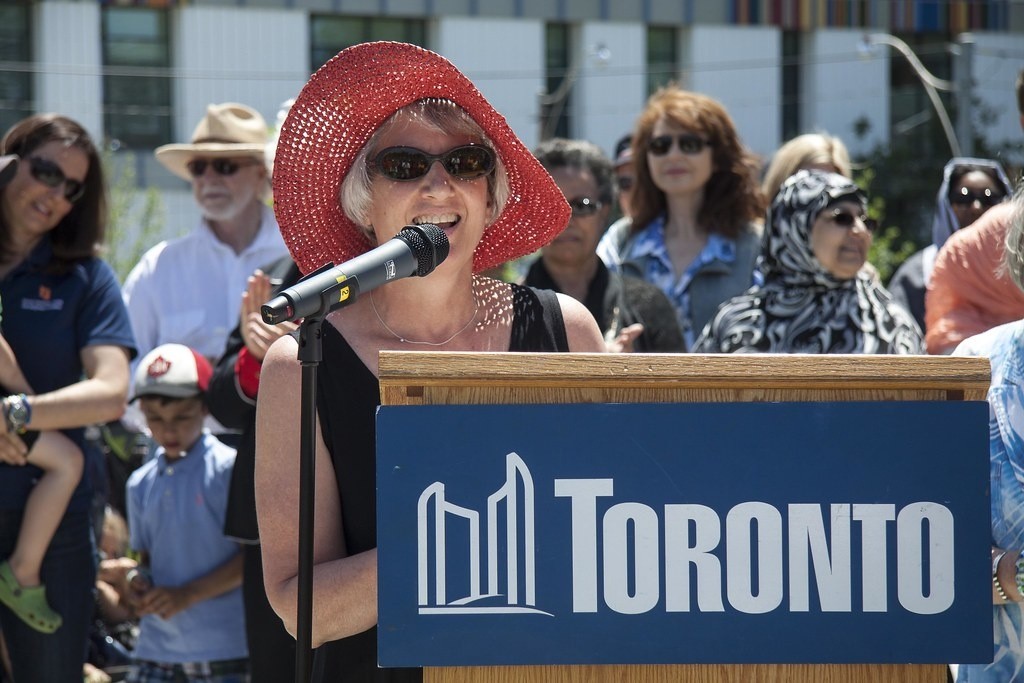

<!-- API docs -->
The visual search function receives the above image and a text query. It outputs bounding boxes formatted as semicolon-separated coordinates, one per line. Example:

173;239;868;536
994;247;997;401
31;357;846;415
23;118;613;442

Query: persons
256;41;606;682
118;101;313;683
1;114;141;683
945;173;1024;682
883;157;1012;338
752;132;856;241
687;169;926;357
517;138;681;352
595;87;764;353
922;67;1024;356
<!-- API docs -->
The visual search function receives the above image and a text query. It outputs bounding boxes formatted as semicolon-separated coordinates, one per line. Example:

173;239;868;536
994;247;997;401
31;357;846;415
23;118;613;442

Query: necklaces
368;289;480;345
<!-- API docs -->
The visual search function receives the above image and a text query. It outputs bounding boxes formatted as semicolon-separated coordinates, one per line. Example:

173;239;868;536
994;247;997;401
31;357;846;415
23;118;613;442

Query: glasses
817;208;878;232
363;144;498;183
644;133;715;157
615;176;635;191
565;195;607;216
949;189;1006;206
17;154;86;205
185;156;266;178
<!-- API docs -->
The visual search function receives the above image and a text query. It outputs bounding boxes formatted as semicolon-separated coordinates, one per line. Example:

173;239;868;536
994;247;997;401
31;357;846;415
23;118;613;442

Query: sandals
0;560;63;636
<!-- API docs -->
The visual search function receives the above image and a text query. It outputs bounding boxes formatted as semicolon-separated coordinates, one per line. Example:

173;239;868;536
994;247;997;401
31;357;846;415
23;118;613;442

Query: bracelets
991;549;1010;605
2;395;31;435
1014;546;1024;596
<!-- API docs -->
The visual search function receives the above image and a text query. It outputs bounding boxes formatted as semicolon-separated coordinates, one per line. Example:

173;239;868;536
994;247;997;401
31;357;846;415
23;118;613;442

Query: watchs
8;394;27;430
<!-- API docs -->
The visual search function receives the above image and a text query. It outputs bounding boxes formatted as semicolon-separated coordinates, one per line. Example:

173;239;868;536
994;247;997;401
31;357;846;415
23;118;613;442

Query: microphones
260;224;450;324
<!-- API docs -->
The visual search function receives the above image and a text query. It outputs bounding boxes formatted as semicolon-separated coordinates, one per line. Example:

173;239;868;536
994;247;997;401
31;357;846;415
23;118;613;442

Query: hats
271;43;573;282
154;102;274;182
126;343;214;406
